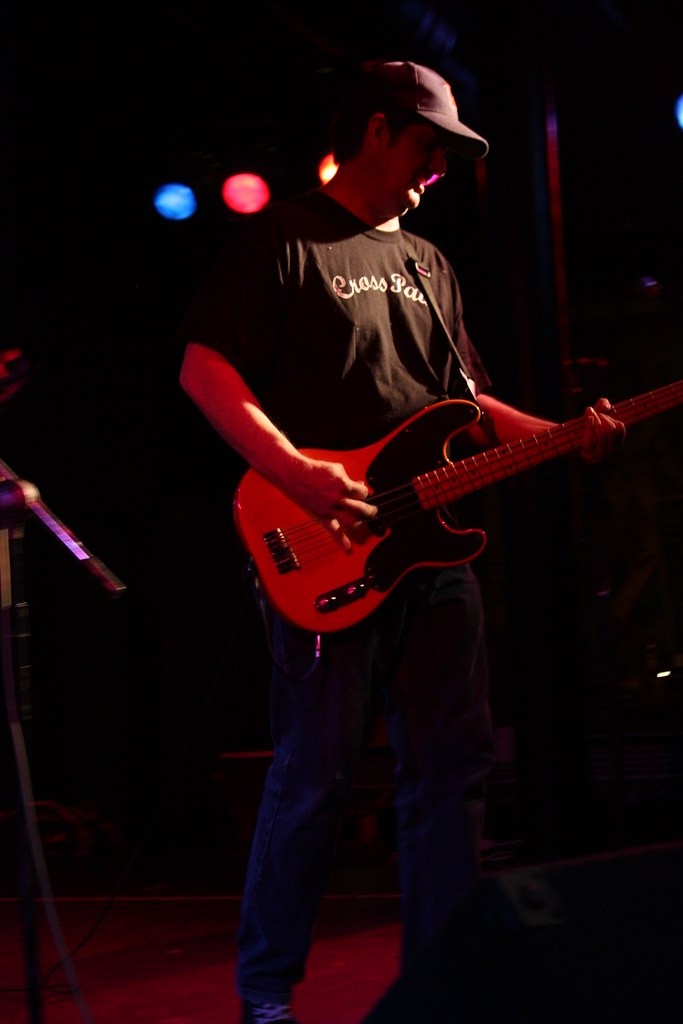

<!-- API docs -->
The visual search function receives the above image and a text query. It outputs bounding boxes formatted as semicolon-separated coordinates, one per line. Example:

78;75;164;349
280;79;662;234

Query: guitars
231;377;682;634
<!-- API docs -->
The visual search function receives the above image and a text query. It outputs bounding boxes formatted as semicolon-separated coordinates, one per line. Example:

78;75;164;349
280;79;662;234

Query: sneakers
243;1000;299;1024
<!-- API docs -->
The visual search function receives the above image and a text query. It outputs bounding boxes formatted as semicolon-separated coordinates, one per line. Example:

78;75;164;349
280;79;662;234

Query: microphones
633;276;683;311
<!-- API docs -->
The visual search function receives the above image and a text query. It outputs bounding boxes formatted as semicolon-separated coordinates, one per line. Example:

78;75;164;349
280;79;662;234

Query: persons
181;60;628;1024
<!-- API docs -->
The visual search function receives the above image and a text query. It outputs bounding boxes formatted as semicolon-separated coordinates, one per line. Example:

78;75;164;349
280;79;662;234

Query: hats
340;60;490;160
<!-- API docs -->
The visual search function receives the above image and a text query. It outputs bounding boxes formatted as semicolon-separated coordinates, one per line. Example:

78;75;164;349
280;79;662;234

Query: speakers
361;841;683;1024
